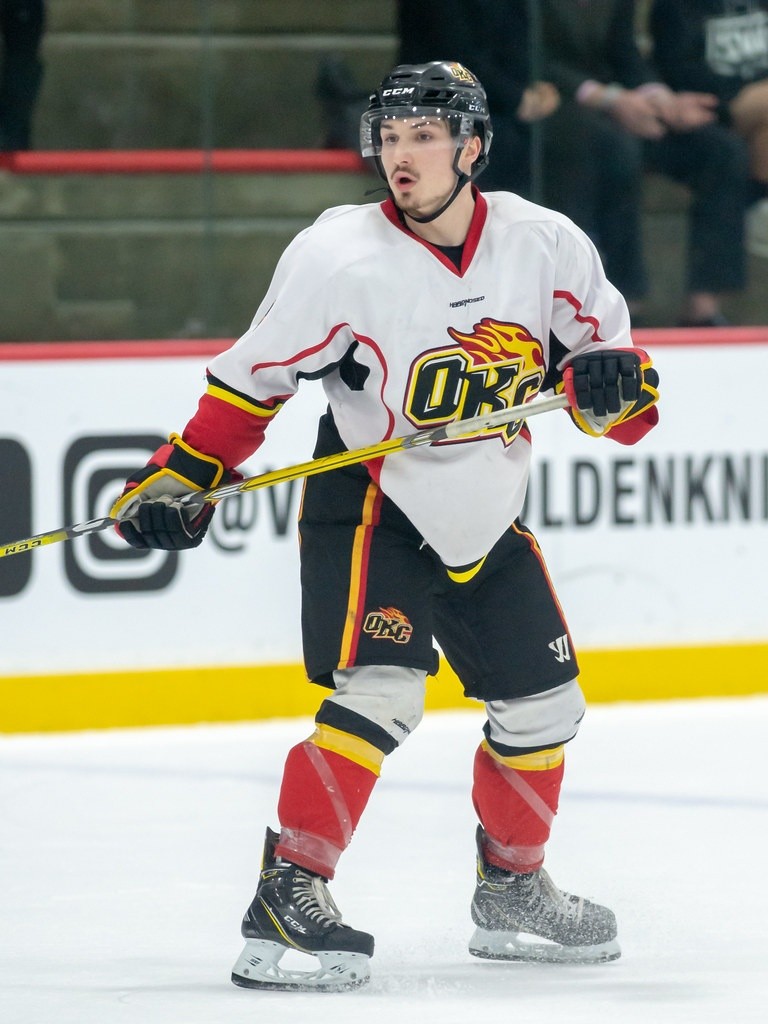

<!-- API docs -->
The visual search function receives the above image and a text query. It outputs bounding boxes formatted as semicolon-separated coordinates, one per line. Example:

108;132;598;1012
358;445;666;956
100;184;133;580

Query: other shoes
630;313;651;327
679;316;728;327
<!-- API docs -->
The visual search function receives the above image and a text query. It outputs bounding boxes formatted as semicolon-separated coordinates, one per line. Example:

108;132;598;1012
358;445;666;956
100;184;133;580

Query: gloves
562;347;659;438
109;433;244;551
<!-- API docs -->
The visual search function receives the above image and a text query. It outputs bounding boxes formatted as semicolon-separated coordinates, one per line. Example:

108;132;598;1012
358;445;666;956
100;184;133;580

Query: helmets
359;59;494;165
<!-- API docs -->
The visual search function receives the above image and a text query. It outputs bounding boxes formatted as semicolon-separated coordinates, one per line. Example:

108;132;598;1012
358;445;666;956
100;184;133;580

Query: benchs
0;147;695;321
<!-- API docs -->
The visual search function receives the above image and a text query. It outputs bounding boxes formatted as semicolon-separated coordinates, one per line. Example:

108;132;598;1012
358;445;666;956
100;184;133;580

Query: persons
106;57;660;994
397;0;768;332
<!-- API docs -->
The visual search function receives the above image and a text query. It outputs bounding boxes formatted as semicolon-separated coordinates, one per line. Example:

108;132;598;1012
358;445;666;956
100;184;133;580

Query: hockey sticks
0;362;642;559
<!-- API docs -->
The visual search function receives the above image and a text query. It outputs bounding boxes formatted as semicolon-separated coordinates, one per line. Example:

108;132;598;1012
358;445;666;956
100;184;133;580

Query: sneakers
231;826;375;992
468;823;621;963
